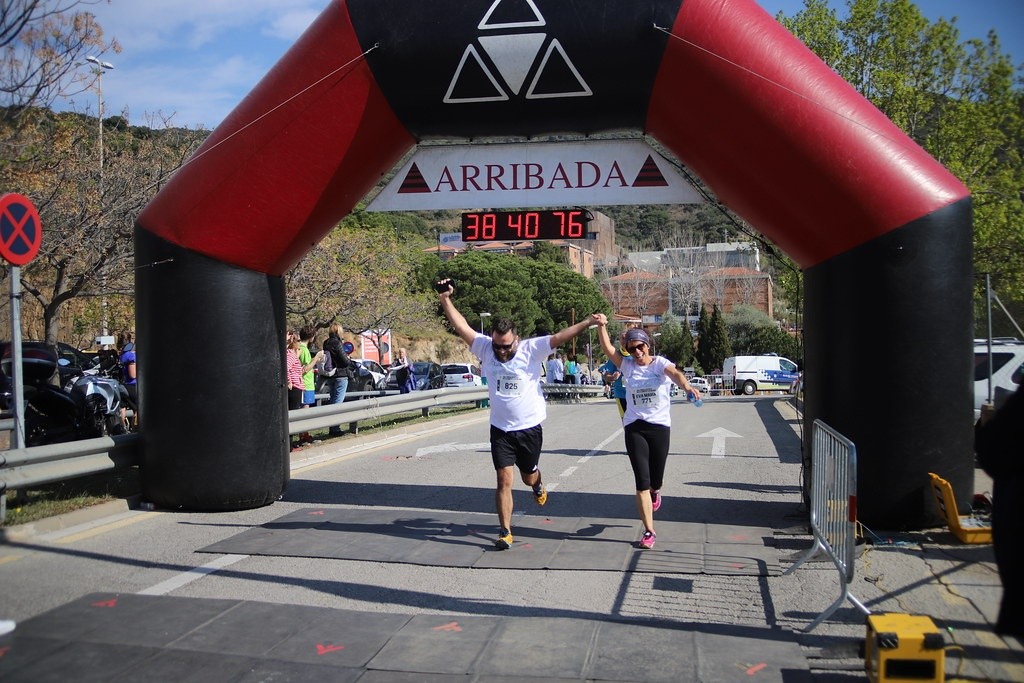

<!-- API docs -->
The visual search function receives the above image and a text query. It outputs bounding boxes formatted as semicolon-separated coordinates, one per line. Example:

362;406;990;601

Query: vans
722;352;804;394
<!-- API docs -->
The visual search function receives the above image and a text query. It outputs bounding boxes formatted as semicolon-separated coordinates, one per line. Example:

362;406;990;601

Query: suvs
441;364;488;387
974;337;1024;467
382;362;447;393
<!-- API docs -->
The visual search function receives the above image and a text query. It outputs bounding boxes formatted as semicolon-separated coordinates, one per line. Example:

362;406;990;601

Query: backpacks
317;350;337;377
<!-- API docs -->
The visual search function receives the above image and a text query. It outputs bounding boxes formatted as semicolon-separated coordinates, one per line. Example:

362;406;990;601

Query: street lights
653;333;661;355
480;312;491;334
589;325;598;385
86;55;114;350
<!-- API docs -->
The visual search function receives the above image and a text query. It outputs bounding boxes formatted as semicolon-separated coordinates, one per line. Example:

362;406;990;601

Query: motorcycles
1;346;130;446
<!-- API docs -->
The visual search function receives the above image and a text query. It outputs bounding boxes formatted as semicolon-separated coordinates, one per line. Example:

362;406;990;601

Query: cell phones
435;279;456;294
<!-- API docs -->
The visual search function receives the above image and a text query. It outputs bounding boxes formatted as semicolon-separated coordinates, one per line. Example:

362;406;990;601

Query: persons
387;348;415;394
546;352;581;398
592;313;700;549
603;331;627;421
285;319;362;451
437;278;607;548
118;332;136;427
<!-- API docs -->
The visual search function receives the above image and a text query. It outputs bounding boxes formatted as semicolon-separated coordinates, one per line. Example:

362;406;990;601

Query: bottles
688;392;703;407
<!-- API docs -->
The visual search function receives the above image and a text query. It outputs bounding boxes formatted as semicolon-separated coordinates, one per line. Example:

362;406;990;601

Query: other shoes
329;430;346;437
298;438;321;447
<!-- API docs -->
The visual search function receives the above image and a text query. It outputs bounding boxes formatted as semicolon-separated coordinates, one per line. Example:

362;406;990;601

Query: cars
688;377;709;393
309;350;375;401
0;339;135;418
350;358;387;388
710;383;722;396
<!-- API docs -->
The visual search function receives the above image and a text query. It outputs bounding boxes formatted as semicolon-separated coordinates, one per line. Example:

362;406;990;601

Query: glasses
493;340;515;349
295;339;301;342
626;342;644;354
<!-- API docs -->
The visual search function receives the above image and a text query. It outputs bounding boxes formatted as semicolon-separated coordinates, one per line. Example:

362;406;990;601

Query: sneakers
495;530;513;549
650;488;662;512
533;469;547;506
639;529;656;548
